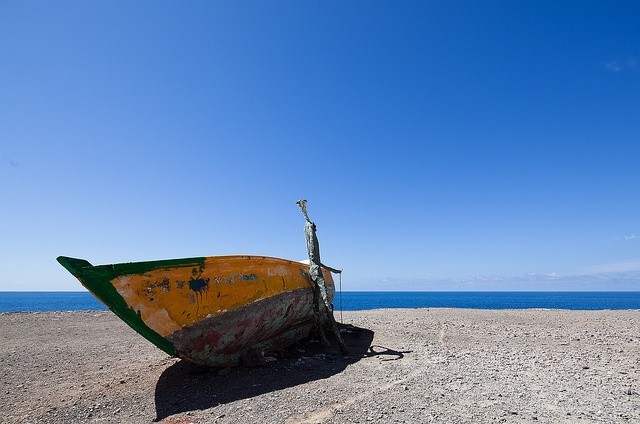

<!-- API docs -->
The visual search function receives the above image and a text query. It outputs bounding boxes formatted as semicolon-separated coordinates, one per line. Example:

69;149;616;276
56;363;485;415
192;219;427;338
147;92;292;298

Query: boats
56;255;336;368
